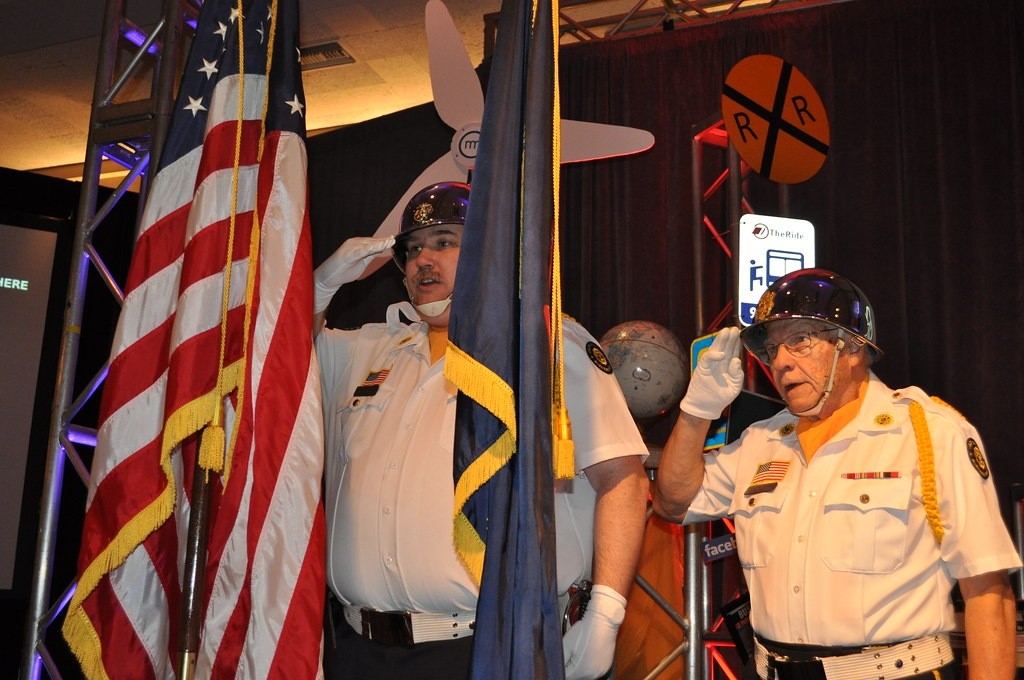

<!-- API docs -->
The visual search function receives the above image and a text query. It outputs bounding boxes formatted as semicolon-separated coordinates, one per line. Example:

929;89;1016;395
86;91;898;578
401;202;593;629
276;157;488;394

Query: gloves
679;327;744;420
562;585;627;680
314;235;396;314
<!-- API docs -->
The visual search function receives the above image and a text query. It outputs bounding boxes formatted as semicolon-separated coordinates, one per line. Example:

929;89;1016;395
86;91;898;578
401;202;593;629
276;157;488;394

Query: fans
355;0;656;281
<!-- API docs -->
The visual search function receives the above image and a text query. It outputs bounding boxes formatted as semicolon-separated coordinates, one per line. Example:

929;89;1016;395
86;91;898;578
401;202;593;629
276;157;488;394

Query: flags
62;0;325;680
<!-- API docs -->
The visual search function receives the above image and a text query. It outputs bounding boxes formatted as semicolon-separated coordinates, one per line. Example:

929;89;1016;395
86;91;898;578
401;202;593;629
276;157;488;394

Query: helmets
739;269;888;368
391;182;471;266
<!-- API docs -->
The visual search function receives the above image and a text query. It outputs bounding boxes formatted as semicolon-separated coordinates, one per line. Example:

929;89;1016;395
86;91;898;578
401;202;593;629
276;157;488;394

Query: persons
312;181;650;680
653;268;1024;680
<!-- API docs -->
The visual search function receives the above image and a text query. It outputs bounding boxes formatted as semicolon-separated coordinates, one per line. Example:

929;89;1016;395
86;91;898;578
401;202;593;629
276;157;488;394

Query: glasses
757;328;839;364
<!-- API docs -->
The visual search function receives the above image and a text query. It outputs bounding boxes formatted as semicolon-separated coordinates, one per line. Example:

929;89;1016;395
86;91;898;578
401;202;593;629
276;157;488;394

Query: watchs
585;599;626;625
